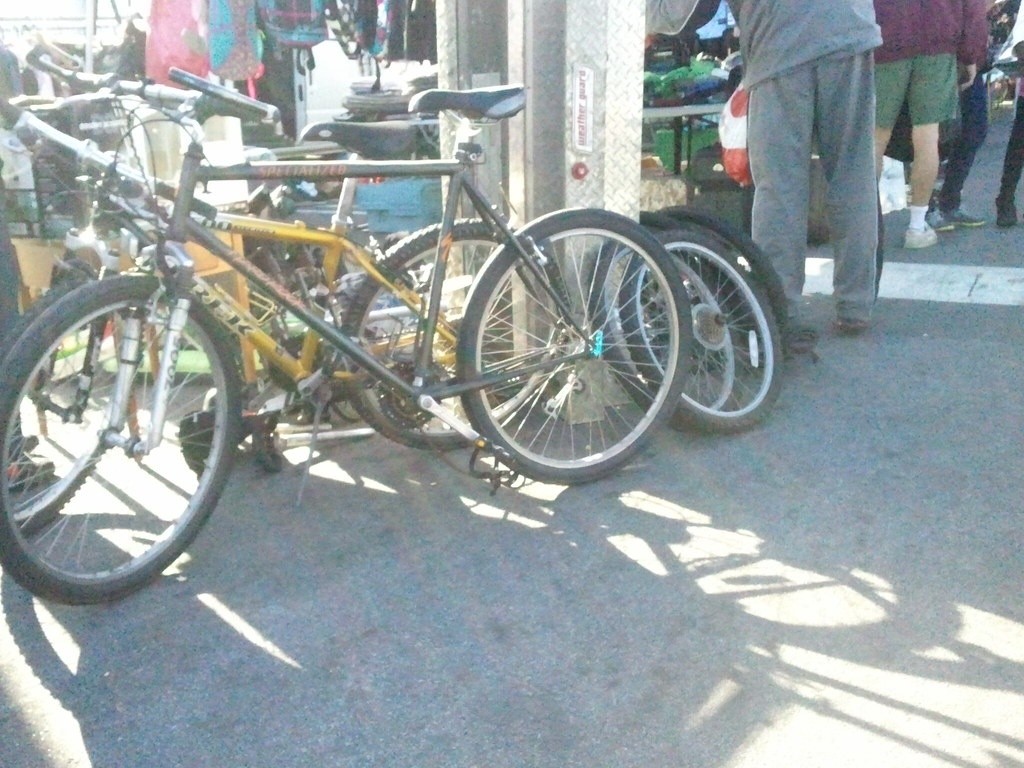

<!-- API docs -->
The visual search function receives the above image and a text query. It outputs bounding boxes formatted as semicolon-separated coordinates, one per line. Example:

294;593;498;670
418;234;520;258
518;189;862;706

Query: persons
647;0;883;354
872;0;1024;248
0;202;56;493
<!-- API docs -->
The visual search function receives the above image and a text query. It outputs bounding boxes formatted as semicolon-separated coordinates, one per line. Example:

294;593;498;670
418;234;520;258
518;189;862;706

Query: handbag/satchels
718;79;754;188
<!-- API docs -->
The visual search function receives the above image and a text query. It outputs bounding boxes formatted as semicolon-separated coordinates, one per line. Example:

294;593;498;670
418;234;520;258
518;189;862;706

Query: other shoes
941;207;985;227
905;220;937;249
996;204;1017;226
22;434;39;454
837;316;867;333
18;454;55;486
927;209;955;231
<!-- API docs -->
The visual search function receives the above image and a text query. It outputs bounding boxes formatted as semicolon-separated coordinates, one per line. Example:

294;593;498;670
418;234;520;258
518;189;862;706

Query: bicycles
1;33;791;608
